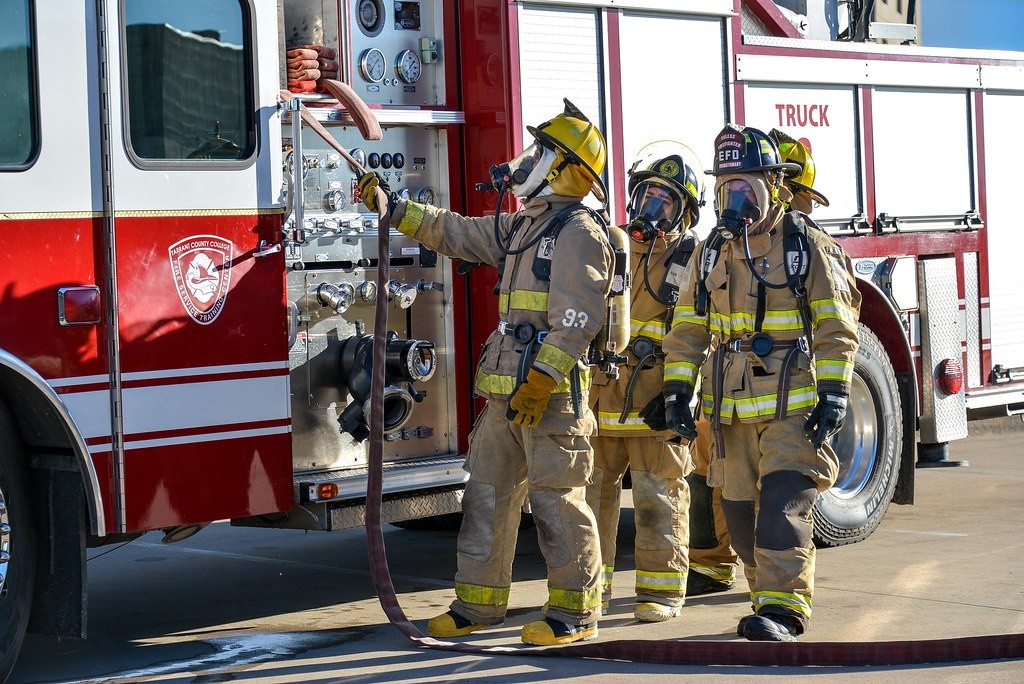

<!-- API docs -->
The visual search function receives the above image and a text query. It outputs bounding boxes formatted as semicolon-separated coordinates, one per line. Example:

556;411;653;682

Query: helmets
628;154;699;211
776;142;830;207
703;124;802;179
527;113;606;182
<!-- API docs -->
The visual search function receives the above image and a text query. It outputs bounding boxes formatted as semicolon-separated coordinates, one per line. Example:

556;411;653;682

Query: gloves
355;166;397;217
666;394;698;442
506;368;557;428
804;392;848;449
638;393;668;431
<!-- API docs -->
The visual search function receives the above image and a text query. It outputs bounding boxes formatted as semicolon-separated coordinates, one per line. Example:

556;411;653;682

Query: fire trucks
0;0;1023;684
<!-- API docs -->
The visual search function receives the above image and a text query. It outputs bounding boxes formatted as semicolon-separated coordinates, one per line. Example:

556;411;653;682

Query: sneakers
742;611;797;641
634;603;682;622
542;600;609;616
685;568;737;596
521;618;598;646
428;610;503;637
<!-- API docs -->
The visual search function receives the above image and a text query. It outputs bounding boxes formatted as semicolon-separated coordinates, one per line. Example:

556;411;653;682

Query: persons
349;113;617;649
589;121;861;639
